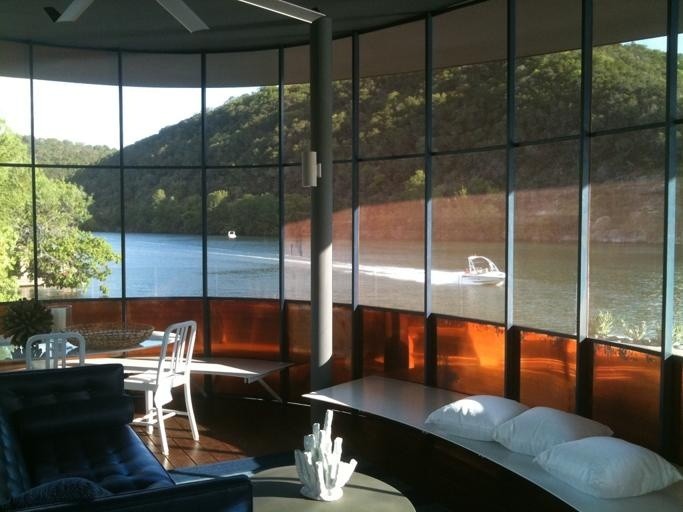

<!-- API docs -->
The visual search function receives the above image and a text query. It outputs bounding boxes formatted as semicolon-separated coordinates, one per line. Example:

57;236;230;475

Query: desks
0;330;180;372
57;354;296;403
301;375;683;512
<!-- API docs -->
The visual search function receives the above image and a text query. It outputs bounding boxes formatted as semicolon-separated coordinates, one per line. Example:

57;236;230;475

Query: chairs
25;332;86;370
122;320;200;455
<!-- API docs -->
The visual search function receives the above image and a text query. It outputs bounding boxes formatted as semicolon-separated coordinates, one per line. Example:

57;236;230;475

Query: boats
458;255;506;285
228;230;237;238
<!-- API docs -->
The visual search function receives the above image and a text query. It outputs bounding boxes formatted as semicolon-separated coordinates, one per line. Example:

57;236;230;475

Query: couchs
0;363;253;512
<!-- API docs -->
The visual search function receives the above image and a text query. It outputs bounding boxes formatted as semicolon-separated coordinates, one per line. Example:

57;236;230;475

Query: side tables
248;464;417;512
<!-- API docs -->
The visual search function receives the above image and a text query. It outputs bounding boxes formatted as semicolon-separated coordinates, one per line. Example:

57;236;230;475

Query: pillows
422;394;682;500
7;476;115;510
